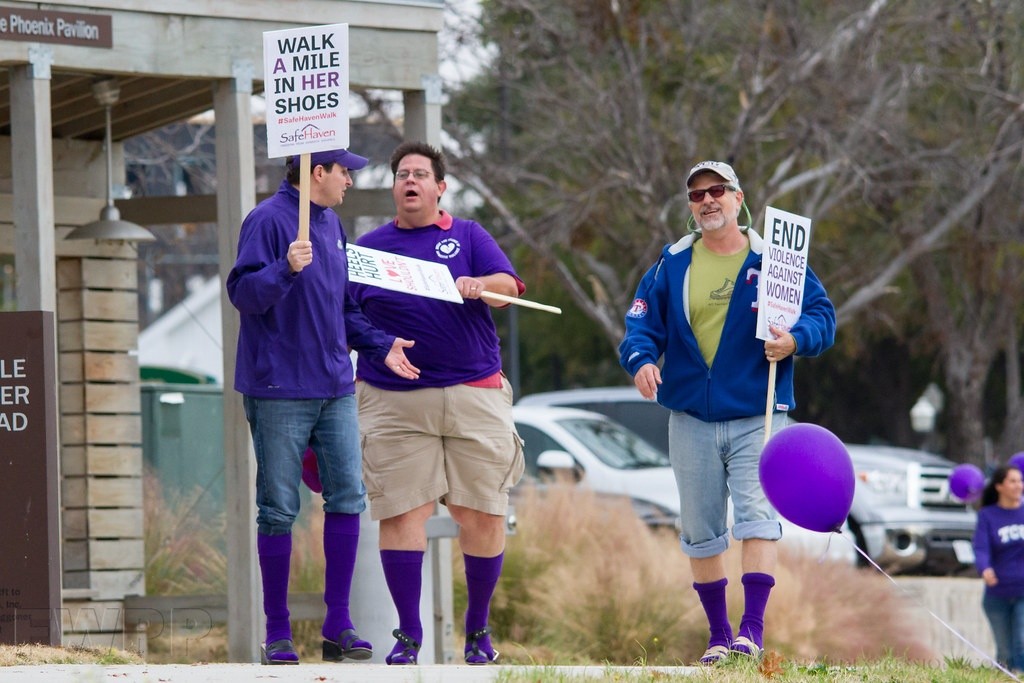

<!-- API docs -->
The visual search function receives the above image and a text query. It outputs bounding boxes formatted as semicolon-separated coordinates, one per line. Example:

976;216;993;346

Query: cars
514;384;674;454
838;441;983;575
508;404;862;572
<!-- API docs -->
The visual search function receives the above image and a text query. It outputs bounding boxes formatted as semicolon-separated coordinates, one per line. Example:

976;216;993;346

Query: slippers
700;636;765;666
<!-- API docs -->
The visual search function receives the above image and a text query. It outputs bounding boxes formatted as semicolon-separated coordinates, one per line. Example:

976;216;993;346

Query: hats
686;161;739;185
287;149;369;170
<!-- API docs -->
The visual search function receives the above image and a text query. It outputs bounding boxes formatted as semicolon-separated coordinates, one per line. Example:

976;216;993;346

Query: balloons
947;462;986;500
1006;451;1024;473
758;422;856;533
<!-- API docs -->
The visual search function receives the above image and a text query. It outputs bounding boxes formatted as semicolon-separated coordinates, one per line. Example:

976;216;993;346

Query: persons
225;148;421;665
348;139;526;665
971;465;1024;672
616;160;836;665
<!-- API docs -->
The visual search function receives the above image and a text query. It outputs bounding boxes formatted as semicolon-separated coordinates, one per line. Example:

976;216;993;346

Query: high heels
385;629;420;665
322;630;372;662
259;640;300;665
465;627;499;665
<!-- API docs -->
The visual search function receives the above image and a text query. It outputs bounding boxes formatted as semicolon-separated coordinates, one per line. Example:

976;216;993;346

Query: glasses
688;185;736;201
397;169;435;180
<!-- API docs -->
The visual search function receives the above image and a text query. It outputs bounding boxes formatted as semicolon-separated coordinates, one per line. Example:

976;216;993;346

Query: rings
769;351;773;356
471;287;477;291
